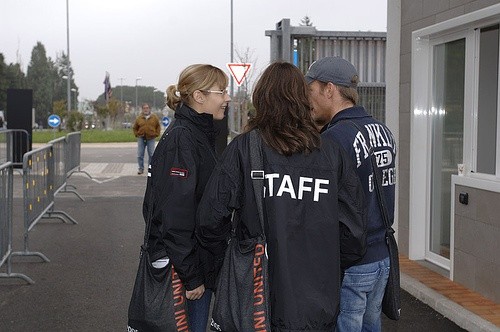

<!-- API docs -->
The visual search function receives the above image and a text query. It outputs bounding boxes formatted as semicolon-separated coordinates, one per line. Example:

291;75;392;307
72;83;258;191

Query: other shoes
138;171;143;174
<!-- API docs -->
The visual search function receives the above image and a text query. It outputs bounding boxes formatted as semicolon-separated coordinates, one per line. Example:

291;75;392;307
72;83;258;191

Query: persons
128;64;231;332
132;104;161;175
196;61;368;332
304;56;397;332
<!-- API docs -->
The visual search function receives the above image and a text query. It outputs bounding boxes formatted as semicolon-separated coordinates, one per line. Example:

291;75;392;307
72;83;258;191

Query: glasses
199;89;228;96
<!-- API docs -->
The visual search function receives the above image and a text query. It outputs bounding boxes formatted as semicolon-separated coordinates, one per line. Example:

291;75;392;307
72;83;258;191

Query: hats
304;57;359;89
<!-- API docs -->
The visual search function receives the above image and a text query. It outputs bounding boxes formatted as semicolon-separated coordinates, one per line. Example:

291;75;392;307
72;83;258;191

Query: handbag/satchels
210;233;271;332
382;227;402;321
126;245;192;332
